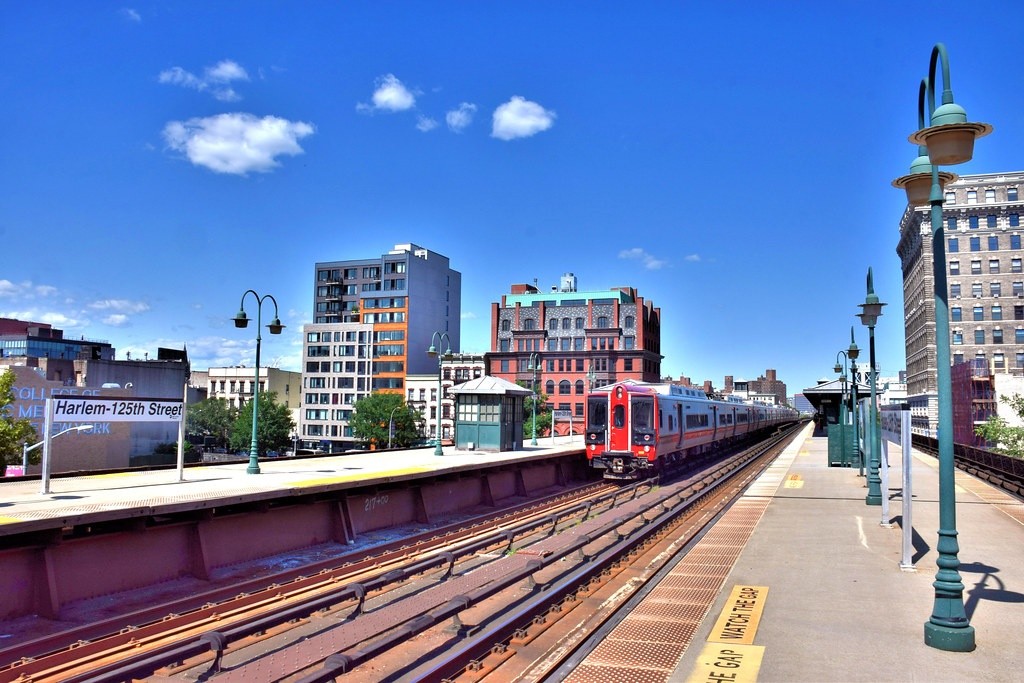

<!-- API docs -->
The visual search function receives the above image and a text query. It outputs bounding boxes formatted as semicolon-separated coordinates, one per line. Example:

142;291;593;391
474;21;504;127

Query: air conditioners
977;295;981;298
956;331;962;334
956;295;961;298
994;293;998;297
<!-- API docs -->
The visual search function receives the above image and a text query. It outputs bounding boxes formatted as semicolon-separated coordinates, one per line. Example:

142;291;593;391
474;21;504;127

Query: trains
584;379;799;481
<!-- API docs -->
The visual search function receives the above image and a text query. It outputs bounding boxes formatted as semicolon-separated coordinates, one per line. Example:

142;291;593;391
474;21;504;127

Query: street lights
833;350;850;422
586;365;596;394
839;373;846;405
230;289;287;475
528;353;544;446
389;406;402;449
845;325;863;469
23;424;93;477
889;43;996;655
424;331;454;456
855;266;885;506
